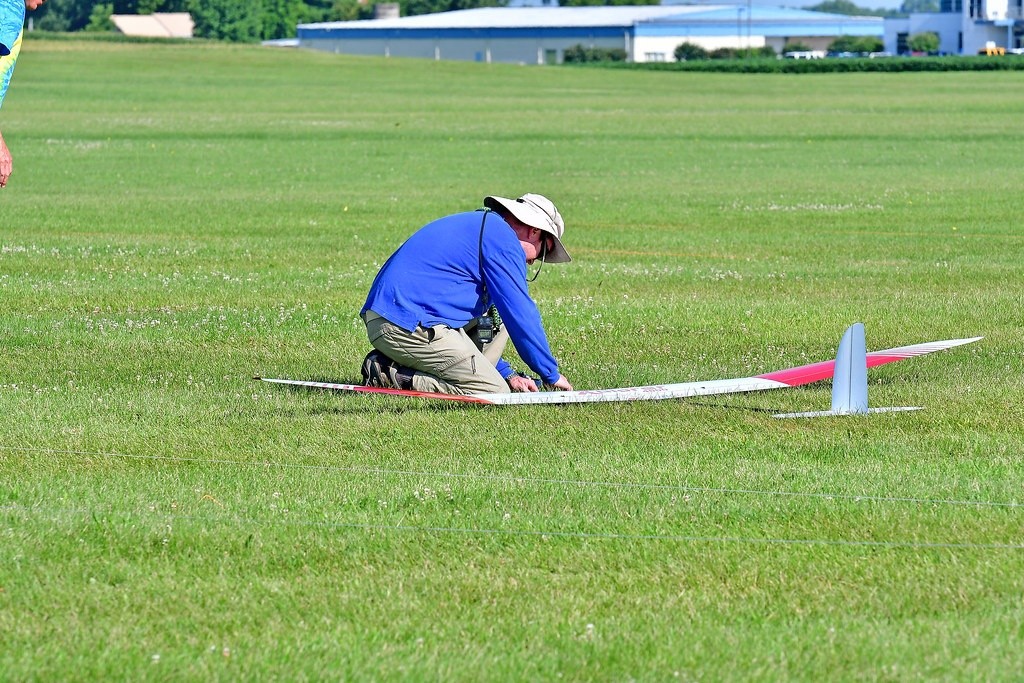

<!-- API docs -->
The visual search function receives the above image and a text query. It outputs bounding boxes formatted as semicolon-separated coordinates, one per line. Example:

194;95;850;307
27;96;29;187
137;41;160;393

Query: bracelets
507;373;517;380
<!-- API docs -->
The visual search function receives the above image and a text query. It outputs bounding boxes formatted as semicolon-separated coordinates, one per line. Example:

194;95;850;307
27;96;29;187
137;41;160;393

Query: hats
483;194;571;263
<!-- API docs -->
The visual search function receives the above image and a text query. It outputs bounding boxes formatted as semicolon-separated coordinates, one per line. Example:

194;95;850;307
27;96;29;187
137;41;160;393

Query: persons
361;193;573;395
0;0;45;189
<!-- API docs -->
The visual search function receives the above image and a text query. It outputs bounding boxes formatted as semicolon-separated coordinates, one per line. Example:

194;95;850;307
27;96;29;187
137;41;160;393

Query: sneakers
361;347;400;391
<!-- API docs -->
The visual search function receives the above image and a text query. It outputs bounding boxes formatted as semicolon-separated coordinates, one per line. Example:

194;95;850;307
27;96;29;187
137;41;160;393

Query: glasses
536;230;549;259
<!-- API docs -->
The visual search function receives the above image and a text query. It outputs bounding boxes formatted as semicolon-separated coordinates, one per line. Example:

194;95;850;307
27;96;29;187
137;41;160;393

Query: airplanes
254;320;987;421
